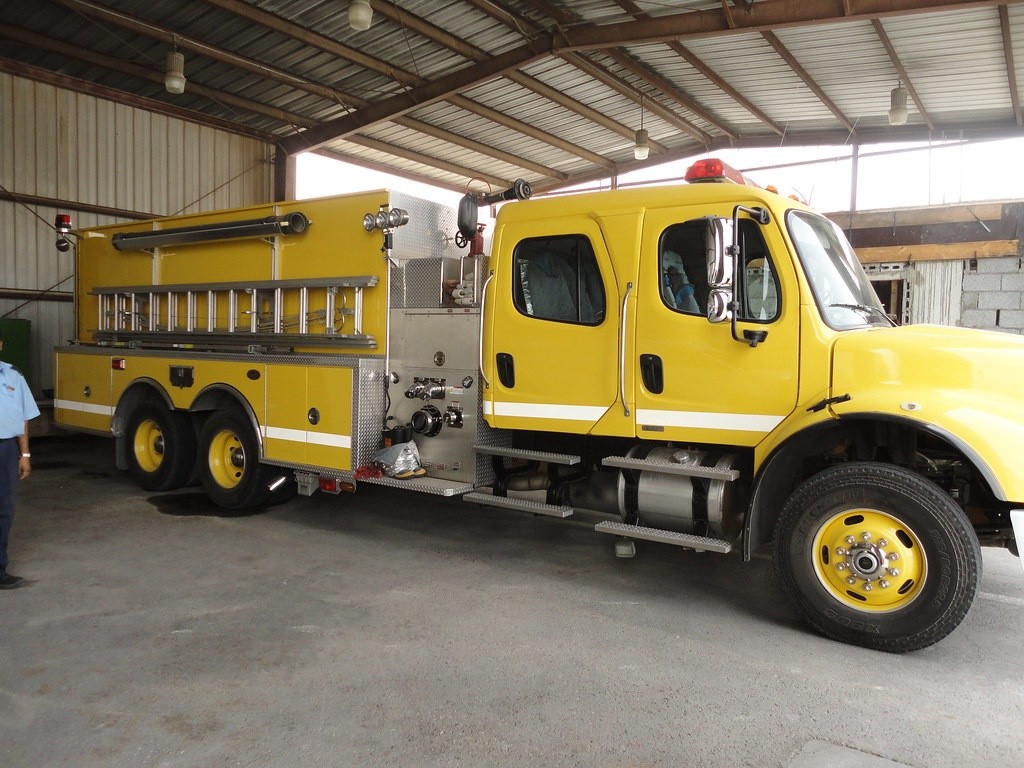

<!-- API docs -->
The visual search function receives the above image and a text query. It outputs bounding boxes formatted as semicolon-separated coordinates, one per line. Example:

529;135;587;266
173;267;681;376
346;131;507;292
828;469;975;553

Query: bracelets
19;453;31;457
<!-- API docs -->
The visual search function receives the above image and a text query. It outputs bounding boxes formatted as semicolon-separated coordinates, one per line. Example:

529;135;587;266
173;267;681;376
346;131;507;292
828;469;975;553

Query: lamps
345;0;374;32
887;82;908;126
163;36;187;95
633;94;650;161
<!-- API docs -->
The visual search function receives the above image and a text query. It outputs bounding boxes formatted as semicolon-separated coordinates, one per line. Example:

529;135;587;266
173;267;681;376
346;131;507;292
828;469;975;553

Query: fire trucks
48;160;1024;653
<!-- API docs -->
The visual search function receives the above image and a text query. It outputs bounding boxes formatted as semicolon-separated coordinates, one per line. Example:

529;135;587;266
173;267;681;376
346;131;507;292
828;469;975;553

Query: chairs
528;250;706;322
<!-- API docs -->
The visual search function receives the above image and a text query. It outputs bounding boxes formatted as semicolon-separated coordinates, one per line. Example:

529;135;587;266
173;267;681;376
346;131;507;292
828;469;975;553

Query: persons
0;338;41;590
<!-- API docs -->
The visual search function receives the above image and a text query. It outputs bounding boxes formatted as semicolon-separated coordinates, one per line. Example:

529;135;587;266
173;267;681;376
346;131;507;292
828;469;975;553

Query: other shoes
395;465;427;480
0;572;25;589
